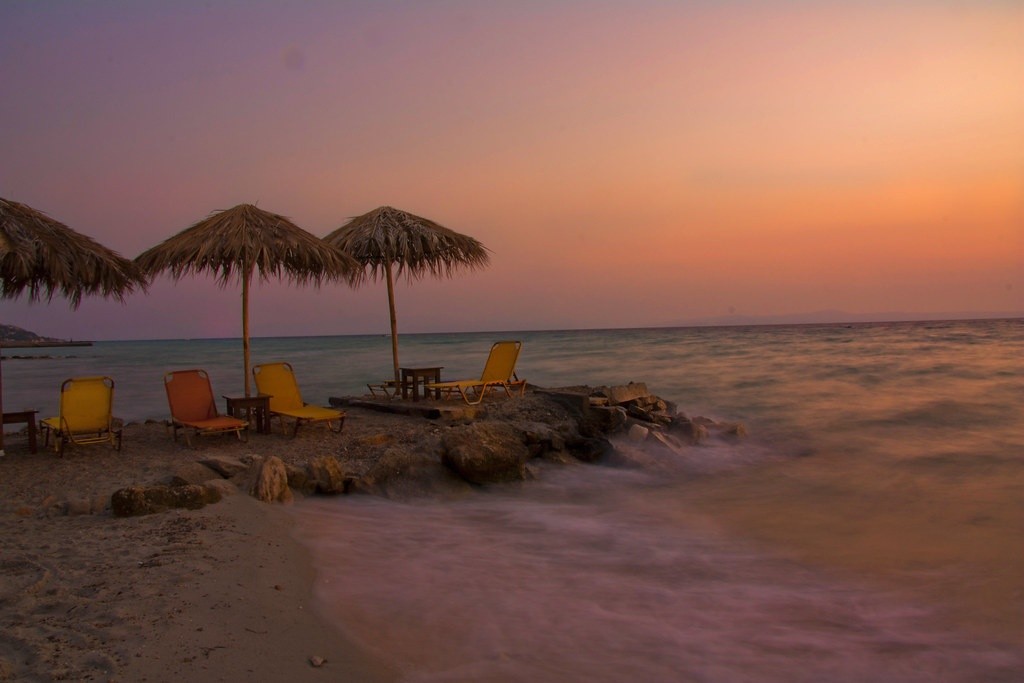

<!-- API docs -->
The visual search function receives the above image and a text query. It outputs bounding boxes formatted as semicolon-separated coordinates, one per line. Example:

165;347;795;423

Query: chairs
424;340;527;406
164;368;250;448
251;360;348;441
41;375;124;456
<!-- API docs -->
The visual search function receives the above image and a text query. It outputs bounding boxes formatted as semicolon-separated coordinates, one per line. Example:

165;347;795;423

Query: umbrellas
132;204;363;428
321;205;491;399
1;195;147;308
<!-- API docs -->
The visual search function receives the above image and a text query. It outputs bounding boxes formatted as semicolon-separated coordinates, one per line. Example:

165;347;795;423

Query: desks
2;408;41;456
398;364;445;402
223;391;275;436
